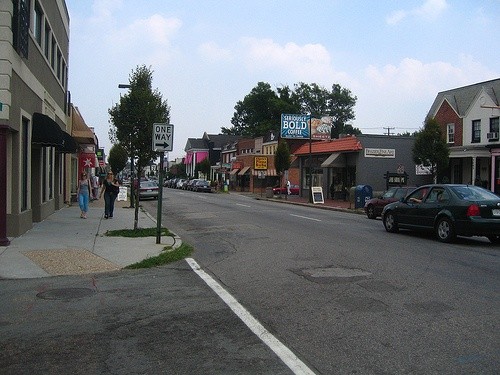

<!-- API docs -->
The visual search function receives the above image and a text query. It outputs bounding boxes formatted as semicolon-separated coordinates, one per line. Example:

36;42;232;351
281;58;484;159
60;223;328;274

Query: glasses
107;173;112;175
82;175;86;177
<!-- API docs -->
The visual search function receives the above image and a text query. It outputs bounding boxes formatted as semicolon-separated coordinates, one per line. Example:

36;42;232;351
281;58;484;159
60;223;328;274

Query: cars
164;179;211;192
133;178;159;199
273;184;300;194
363;184;500;242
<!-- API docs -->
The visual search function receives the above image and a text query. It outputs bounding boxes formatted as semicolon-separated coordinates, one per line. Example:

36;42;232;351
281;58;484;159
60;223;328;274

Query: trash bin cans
265;187;273;198
350;186;357;202
223;183;229;192
357;185;373;208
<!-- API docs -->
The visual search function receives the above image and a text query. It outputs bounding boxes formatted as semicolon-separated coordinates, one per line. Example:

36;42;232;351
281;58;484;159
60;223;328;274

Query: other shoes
83;215;87;219
80;213;83;218
109;217;112;219
104;215;108;219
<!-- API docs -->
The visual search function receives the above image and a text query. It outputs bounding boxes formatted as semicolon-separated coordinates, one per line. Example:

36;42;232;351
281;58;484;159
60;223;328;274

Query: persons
285;180;293;196
76;171;93;219
90;173;99;200
409;193;428;204
100;172;120;219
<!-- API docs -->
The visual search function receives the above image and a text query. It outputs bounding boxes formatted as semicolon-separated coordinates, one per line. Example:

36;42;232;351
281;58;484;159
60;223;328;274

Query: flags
98;161;105;167
78;152;96;169
183;152;193;166
197;152;208;163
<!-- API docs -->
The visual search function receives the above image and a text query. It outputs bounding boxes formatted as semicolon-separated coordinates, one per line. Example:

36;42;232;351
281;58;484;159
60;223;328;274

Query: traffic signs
151;123;173;151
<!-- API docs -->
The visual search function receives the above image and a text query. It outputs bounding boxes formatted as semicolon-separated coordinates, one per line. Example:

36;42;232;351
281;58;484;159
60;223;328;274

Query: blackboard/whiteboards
314;192;323;202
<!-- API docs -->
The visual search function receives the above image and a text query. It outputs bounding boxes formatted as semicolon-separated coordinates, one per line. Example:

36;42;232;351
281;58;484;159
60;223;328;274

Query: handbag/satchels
113;179;120;194
